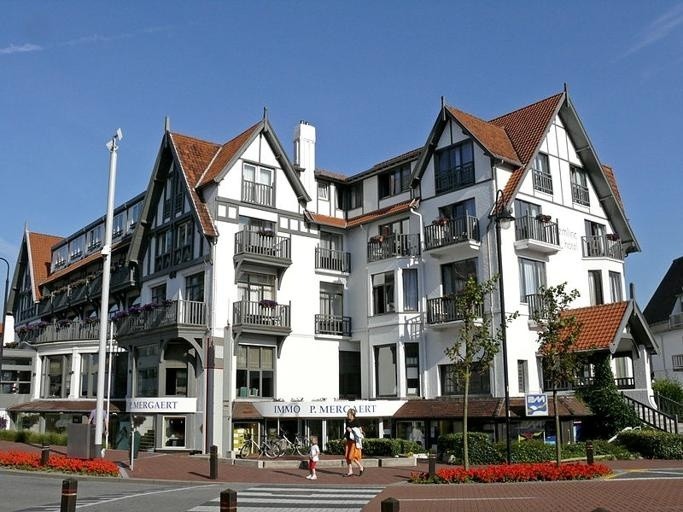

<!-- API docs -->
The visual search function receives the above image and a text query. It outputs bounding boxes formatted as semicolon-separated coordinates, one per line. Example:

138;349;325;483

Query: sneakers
306;474;317;480
343;466;365;477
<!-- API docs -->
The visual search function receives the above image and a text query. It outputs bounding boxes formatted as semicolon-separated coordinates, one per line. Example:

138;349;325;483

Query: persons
108;410;120;450
413;425;425;445
305;436;322;480
86;405;110;440
408;428;415;439
342;408;365;479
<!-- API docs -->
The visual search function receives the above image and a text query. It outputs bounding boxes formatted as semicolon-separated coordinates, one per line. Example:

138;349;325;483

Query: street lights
495;188;516;464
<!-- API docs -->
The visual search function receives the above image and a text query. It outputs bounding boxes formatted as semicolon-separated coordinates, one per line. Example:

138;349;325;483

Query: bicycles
240;427;280;459
272;427;312;457
